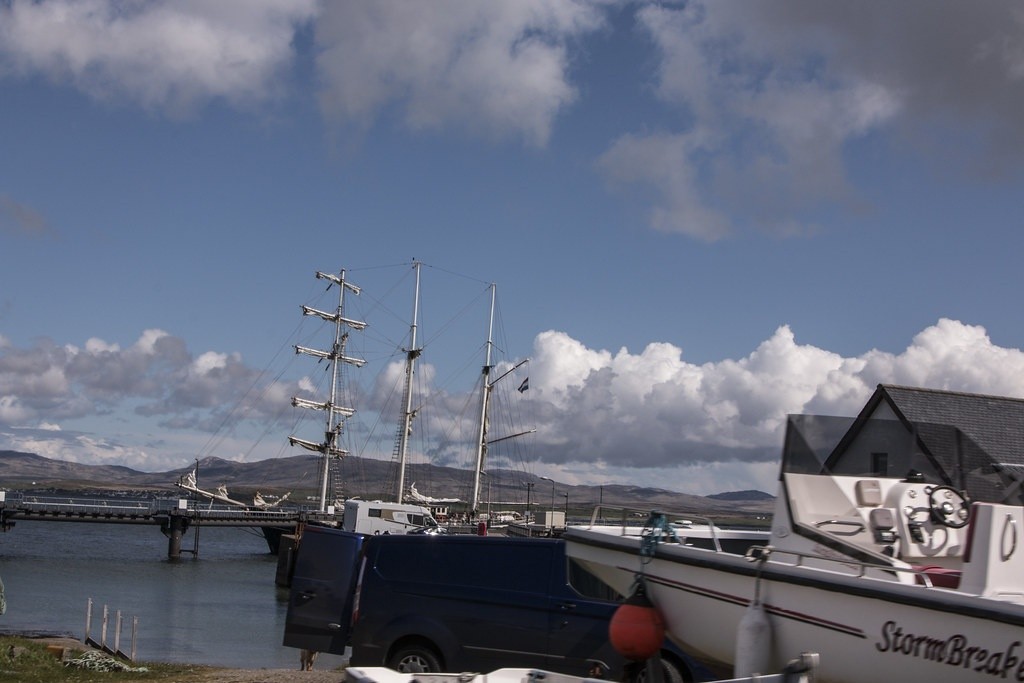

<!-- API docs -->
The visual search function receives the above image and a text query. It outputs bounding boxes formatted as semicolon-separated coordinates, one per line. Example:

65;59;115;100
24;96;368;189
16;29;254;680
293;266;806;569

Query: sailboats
171;257;537;561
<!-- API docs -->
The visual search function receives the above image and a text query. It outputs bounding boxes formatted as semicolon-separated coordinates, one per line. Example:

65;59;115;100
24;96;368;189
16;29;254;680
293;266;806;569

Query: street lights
540;476;554;536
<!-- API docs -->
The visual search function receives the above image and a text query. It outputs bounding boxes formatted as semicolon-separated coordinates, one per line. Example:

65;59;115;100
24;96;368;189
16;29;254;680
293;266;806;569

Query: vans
343;497;449;536
276;525;690;681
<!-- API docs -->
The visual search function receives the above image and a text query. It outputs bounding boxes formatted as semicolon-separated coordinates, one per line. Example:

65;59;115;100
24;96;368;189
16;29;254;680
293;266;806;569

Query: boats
563;471;1024;683
